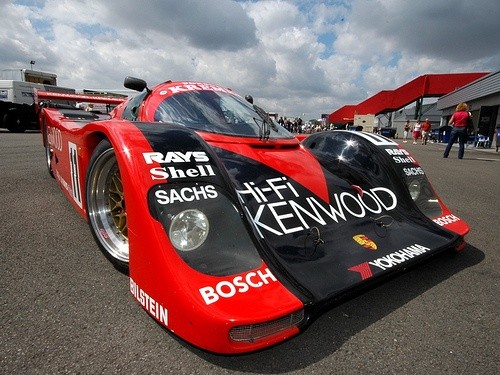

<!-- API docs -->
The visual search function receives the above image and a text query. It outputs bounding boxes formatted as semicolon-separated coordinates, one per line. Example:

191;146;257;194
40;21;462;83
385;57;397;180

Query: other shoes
403;141;407;143
413;142;417;144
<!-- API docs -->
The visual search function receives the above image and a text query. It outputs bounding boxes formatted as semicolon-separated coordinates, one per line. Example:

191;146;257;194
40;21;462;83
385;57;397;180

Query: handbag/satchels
466;112;474;132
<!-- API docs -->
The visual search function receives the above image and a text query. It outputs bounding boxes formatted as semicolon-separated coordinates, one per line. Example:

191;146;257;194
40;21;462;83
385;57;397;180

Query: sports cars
33;77;470;355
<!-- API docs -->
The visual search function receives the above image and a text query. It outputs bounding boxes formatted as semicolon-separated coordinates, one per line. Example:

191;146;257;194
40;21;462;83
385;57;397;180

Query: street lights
30;60;35;70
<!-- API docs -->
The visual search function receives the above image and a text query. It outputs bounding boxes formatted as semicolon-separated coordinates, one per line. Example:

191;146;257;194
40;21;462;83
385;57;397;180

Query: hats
426;119;429;120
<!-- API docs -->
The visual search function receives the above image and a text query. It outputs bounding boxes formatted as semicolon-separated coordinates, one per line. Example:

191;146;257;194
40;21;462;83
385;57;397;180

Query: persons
495;127;500;152
402;118;432;145
442;102;473;159
277;117;351;134
84;102;95;112
